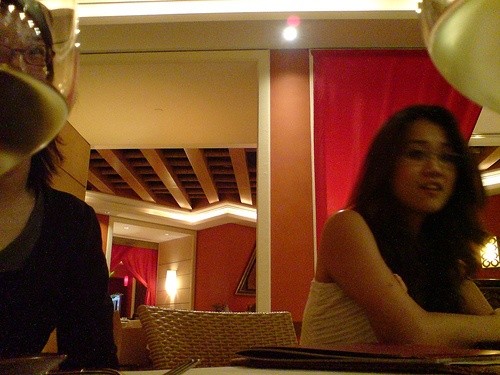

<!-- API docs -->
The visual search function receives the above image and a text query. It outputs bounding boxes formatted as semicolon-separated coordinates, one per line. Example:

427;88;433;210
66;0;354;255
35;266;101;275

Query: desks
0;353;67;375
228;336;500;375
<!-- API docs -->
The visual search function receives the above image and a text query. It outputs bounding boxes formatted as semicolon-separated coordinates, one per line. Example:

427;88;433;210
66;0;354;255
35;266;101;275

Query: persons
300;102;500;349
0;0;119;375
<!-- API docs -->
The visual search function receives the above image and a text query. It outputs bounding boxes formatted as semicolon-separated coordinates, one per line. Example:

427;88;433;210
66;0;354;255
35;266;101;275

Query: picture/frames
235;248;255;297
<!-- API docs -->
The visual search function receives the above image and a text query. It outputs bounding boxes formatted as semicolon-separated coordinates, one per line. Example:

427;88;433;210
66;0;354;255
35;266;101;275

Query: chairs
50;355;203;375
137;304;299;369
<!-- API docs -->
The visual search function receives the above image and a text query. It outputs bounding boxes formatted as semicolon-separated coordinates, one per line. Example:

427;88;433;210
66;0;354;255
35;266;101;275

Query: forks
49;356;205;375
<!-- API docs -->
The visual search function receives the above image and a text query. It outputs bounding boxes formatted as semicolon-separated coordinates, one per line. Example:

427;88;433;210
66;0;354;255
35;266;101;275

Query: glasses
0;43;56;66
400;149;468;170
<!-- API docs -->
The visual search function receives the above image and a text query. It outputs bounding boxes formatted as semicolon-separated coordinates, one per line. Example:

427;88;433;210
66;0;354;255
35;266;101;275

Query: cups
0;0;78;178
418;0;500;112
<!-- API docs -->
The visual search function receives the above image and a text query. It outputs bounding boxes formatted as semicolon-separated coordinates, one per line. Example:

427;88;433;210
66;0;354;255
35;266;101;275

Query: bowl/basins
0;353;69;375
